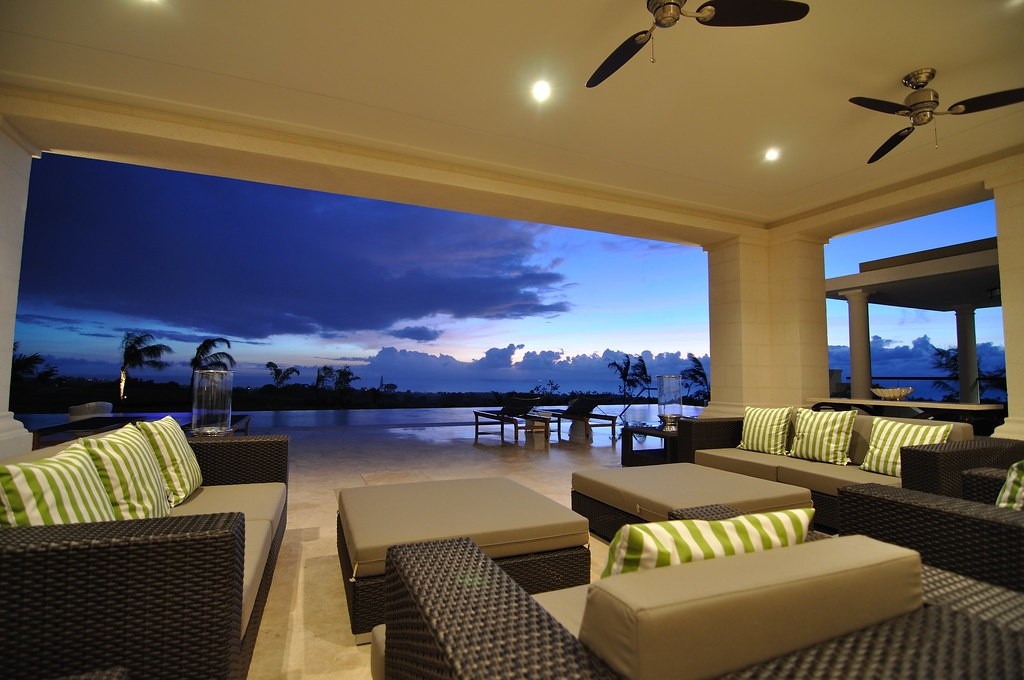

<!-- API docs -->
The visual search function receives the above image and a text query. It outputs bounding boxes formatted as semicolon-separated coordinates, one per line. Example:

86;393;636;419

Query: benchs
180;411;251;436
337;477;592;647
29;416;147;454
572;462;814;541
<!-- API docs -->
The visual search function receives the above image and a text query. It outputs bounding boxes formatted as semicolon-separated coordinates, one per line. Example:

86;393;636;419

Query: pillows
858;417;953;477
136;415;204;508
600;508;817;580
787;409;858;466
0;438;117;528
79;422;171;521
995;461;1024;511
736;405;795;456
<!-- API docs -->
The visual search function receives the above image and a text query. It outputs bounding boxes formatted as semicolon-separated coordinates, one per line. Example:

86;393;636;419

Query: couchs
369;483;1024;680
679;407;1024;538
0;429;288;680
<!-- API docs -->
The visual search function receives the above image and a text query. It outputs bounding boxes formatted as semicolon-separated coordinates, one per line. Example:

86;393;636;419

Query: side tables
621;427;679;468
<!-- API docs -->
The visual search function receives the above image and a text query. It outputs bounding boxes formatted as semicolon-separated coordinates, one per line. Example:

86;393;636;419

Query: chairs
837;468;1024;592
474;395;618;443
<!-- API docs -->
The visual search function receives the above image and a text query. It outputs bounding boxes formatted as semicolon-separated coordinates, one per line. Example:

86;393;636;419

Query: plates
870;388;913;401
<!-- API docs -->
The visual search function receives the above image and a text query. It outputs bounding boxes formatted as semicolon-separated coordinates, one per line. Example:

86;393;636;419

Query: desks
805;397;1004;426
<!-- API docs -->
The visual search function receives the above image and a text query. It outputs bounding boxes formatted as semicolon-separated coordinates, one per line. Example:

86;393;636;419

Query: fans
584;0;810;88
848;68;1024;165
